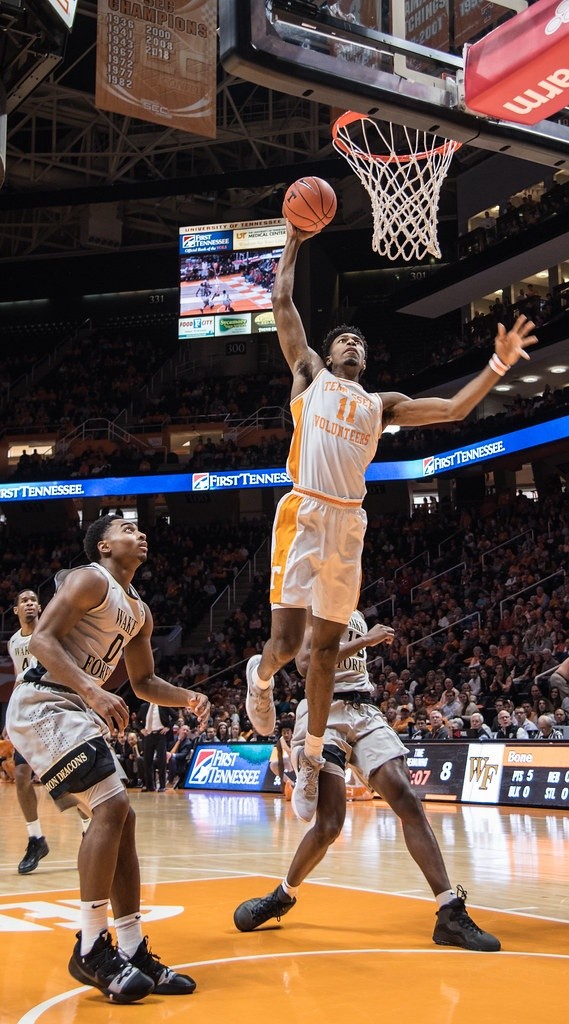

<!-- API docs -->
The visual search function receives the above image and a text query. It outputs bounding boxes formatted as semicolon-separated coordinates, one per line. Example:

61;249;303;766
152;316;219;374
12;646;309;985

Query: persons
6;514;210;1004
0;186;569;952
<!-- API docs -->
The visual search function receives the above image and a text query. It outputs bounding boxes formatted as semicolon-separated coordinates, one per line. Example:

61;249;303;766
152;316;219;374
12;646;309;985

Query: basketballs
283;176;337;231
212;262;220;269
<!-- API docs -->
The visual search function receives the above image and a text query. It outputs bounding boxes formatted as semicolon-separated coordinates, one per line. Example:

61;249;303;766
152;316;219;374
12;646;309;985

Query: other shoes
141;786;147;791
157;787;166;792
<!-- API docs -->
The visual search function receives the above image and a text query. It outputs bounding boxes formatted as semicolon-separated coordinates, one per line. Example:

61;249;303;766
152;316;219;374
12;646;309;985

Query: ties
147;704;155;734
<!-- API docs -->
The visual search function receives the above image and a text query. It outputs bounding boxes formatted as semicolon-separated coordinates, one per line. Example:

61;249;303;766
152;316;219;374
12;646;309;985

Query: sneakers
290;745;326;822
18;836;49;872
246;654;276;737
116;934;196;993
68;930;154;1002
432;885;502;952
234;884;297;932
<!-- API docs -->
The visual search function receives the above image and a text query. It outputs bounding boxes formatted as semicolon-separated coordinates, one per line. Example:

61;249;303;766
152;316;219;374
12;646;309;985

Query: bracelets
485;353;512;388
